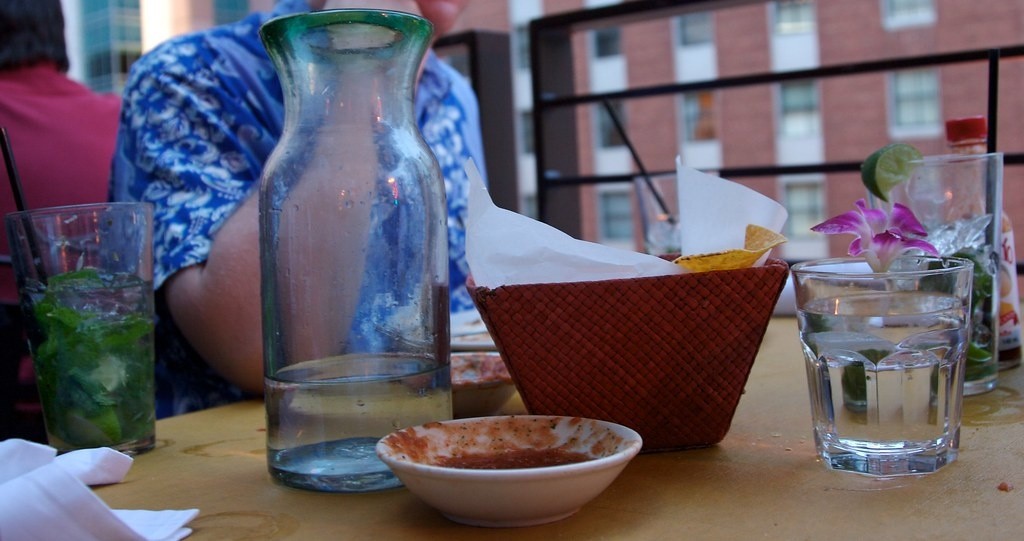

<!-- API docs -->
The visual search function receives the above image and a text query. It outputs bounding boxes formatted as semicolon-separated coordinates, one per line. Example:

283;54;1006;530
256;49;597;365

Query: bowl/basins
449;355;516;417
375;415;643;527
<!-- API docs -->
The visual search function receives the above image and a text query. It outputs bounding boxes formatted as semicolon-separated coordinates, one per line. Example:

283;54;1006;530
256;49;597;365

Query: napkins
0;438;201;541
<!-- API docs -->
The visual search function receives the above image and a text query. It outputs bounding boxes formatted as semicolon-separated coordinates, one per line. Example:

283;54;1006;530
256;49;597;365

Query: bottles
258;7;454;493
944;115;1024;371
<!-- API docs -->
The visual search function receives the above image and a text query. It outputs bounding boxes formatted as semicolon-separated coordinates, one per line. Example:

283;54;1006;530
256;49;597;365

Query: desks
88;313;1024;541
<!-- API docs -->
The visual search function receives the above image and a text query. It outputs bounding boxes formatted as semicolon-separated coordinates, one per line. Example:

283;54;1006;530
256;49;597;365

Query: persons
0;0;121;412
100;0;479;419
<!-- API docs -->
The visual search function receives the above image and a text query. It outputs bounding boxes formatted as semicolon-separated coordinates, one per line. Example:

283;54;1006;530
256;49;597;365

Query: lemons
860;144;923;202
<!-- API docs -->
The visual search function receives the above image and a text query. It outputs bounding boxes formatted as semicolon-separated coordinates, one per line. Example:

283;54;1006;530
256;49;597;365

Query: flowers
810;198;945;271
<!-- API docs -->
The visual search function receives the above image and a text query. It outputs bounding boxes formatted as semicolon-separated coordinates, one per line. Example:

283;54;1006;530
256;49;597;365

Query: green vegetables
27;263;154;452
916;249;1000;388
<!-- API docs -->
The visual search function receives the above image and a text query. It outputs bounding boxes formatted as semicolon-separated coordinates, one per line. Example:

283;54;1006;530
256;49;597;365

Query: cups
3;201;157;456
887;151;1003;397
790;253;974;480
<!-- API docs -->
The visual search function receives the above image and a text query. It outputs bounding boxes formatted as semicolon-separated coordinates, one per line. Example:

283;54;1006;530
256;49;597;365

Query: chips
672;223;788;273
